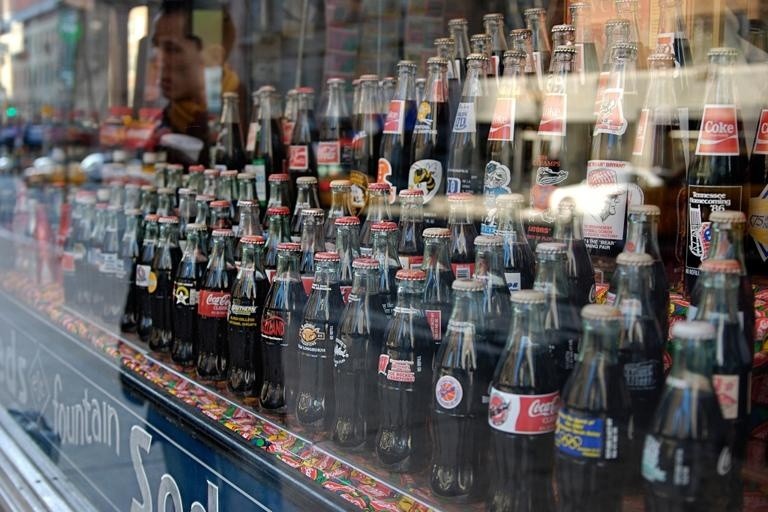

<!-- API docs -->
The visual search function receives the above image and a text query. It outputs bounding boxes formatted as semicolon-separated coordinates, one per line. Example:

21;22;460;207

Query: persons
113;0;306;512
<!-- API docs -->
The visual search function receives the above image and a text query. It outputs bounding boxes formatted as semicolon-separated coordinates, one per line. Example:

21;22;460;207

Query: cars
0;141;151;188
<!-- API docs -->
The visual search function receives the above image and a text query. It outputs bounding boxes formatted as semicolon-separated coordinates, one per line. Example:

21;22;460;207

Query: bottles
7;0;766;512
